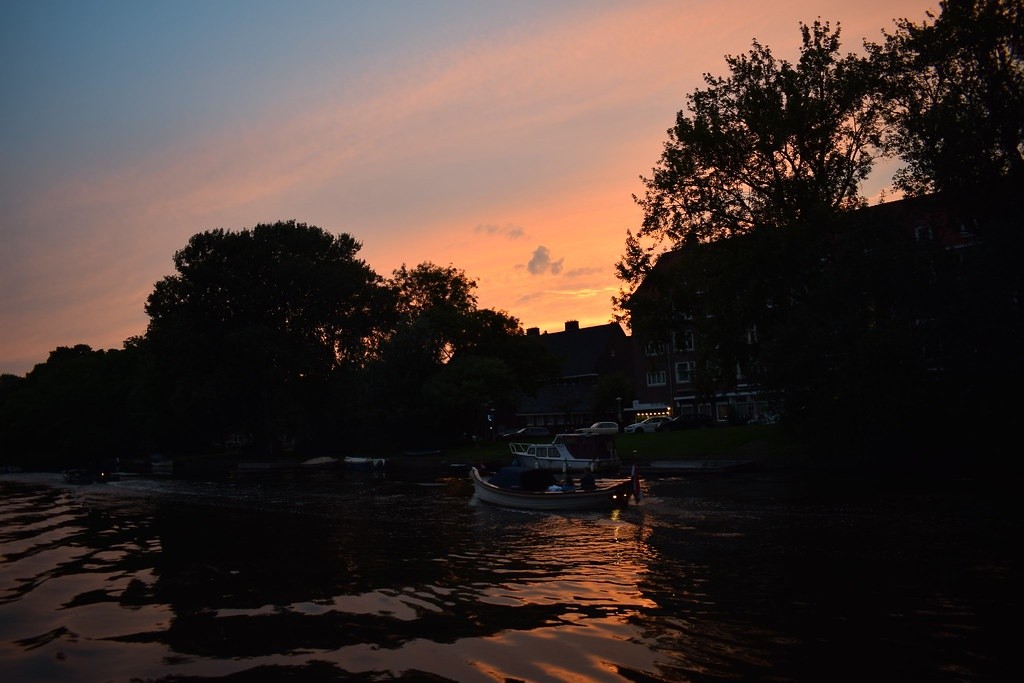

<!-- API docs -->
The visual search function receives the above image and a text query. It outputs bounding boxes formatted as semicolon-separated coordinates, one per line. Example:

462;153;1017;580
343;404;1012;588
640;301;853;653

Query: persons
580;467;595;490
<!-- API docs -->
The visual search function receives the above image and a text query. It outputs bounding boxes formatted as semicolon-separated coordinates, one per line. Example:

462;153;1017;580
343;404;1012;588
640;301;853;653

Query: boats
400;446;445;456
299;455;341;468
509;431;622;475
471;466;635;511
345;455;387;470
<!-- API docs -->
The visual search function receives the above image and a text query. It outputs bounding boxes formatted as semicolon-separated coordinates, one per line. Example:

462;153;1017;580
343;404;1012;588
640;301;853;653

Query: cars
656;413;716;433
624;415;673;434
501;425;549;439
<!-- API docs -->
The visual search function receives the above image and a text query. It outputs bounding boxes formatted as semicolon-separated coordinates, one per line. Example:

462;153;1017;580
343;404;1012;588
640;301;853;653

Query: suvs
574;421;619;437
